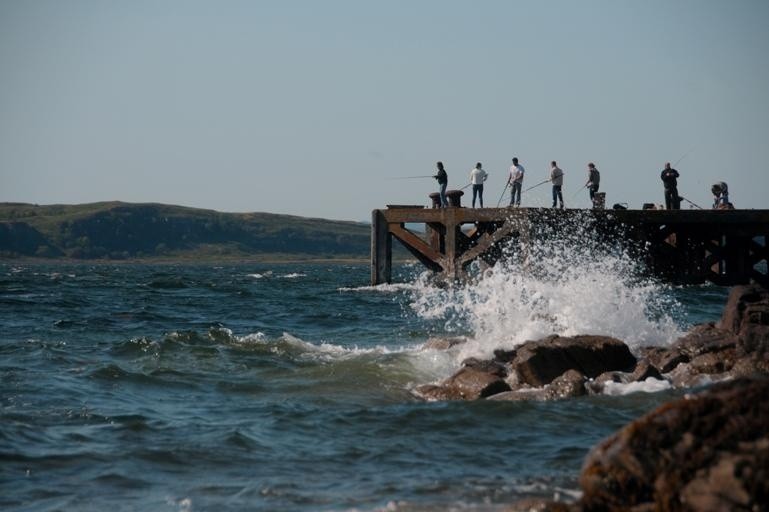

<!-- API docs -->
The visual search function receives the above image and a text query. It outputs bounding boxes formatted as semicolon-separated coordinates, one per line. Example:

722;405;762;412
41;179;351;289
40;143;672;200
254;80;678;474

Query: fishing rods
501;173;564;202
670;153;688;171
384;175;434;179
483;177;511;236
682;197;703;209
458;174;488;191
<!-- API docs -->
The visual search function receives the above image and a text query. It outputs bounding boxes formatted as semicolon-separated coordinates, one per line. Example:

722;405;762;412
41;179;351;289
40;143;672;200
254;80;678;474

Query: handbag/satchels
613;203;627;209
715;203;734;210
643;203;654;209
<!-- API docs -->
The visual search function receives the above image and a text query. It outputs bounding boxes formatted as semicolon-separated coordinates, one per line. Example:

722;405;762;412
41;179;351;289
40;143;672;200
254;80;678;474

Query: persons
544;161;565;210
432;161;450;209
661;163;680;210
506;157;526;208
470;162;489;208
584;163;601;210
711;181;728;210
